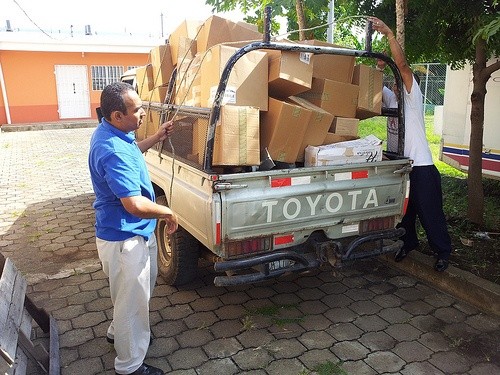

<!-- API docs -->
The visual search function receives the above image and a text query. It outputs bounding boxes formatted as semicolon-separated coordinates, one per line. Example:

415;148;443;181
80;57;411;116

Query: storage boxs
134;15;383;173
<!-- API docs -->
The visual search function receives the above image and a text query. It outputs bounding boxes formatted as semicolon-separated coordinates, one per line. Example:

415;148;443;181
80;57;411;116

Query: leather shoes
395;240;420;262
115;363;167;375
434;253;448;271
105;333;156;347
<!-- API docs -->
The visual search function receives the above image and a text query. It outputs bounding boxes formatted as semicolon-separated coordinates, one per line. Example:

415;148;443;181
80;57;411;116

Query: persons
367;16;452;272
89;82;179;375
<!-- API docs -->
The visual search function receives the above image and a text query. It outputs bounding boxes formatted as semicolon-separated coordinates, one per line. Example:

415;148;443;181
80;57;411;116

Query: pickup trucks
119;6;414;287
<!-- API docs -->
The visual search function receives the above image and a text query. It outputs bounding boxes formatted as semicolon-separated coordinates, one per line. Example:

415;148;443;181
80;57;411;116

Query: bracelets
389;36;395;41
